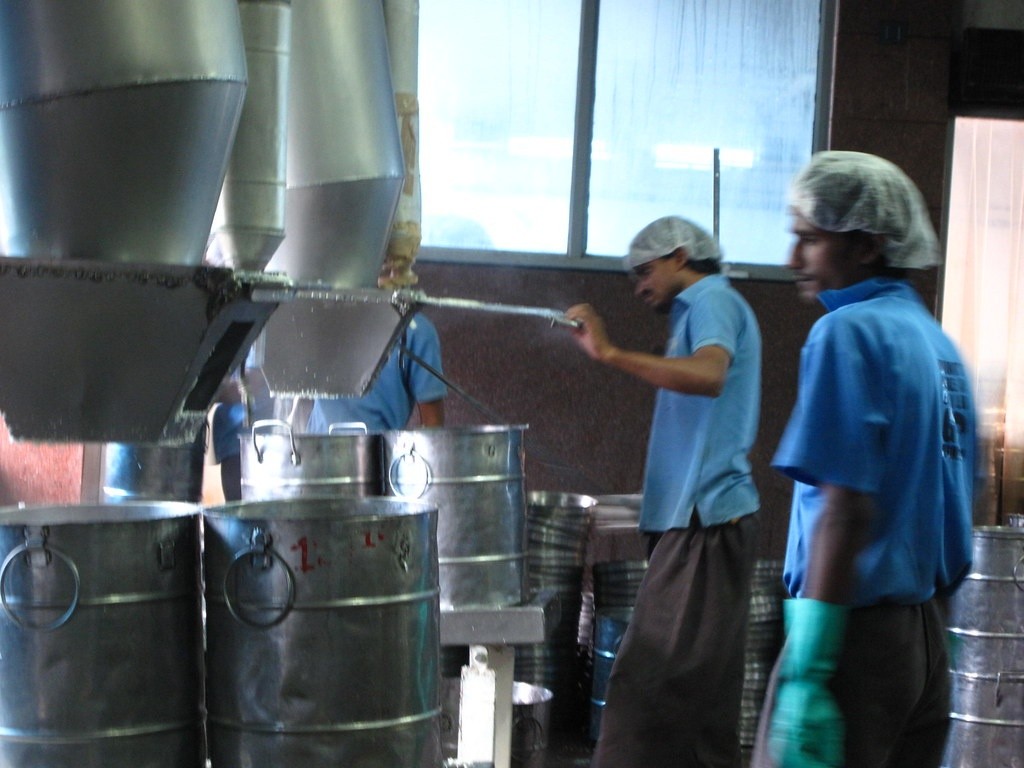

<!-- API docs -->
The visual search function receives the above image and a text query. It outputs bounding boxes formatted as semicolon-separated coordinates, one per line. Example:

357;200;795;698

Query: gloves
766;599;845;767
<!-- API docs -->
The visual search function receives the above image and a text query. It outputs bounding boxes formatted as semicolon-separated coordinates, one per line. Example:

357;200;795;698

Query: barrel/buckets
237;419;382;499
442;677;554;768
0;502;201;768
383;425;527;607
204;497;442;768
98;417;210;505
940;525;1024;768
588;606;633;740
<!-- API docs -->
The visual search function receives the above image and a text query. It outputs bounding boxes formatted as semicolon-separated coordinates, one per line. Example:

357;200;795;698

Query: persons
749;149;977;768
211;313;448;504
563;215;761;768
